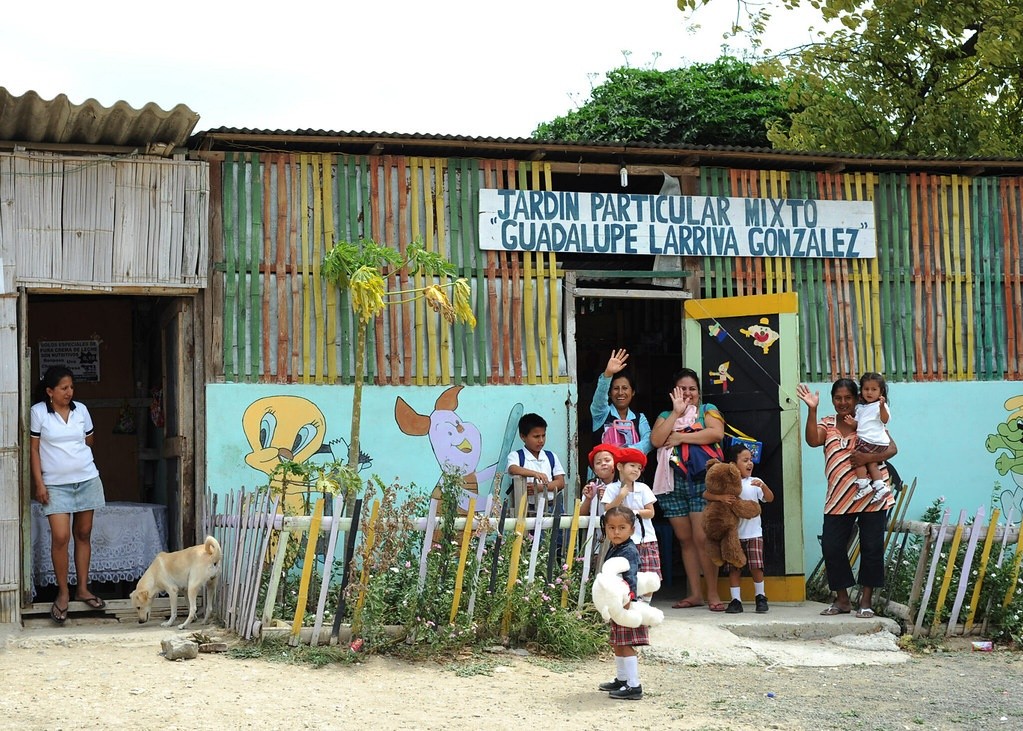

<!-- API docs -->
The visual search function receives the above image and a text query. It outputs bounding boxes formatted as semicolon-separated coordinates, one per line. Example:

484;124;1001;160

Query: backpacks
669;427;725;479
605;419;639;448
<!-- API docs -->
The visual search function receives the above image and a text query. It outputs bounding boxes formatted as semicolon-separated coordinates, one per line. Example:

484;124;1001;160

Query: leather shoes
726;599;743;612
609;684;643;700
756;594;768;611
599;678;627;691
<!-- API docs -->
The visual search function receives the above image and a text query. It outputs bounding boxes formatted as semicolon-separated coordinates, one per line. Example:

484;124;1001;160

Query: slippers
856;607;874;618
51;595;69;623
708;601;725;611
820;602;851;615
672;600;704;608
74;594;105;609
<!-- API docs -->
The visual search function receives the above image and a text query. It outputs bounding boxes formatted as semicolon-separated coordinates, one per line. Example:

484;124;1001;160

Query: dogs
130;536;222;631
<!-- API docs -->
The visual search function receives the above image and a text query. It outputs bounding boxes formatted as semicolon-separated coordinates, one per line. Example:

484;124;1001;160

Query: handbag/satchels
704;410;763;464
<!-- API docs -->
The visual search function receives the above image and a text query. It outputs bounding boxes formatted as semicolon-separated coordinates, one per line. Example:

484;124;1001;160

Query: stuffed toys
592;557;664;630
701;458;762;568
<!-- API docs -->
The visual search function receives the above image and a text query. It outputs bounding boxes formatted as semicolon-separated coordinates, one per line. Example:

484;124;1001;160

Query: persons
796;378;898;618
586;349;652;482
504;413;566;518
598;506;650;700
844;372;891;505
650;367;726;611
580;445;663;605
30;366;105;623
703;445;774;613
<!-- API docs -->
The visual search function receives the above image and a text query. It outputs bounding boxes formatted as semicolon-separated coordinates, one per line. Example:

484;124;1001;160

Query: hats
588;443;618;470
614;449;647;470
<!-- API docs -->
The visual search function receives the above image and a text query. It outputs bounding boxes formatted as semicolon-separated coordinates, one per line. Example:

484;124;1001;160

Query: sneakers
871;484;891;505
852;484;873;501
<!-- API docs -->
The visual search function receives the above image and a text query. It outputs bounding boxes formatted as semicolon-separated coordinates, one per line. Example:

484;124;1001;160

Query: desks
30;501;169;599
